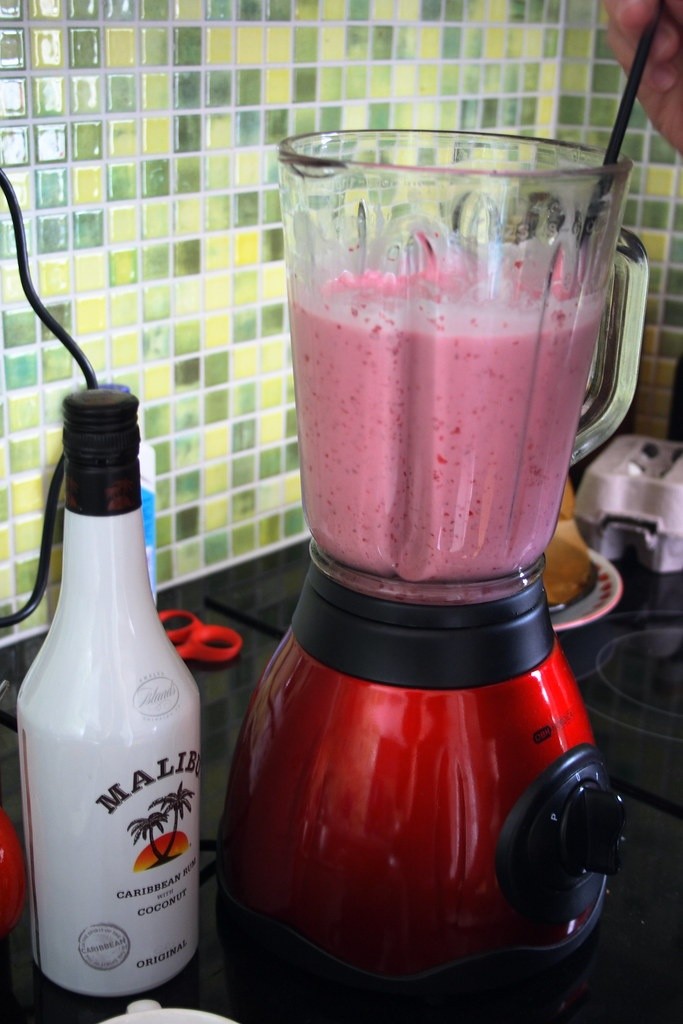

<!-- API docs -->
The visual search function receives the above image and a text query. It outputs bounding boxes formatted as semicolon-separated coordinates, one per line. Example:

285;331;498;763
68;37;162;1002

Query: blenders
210;127;647;978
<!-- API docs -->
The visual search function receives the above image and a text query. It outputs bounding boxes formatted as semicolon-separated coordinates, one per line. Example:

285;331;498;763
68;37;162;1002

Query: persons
606;0;683;158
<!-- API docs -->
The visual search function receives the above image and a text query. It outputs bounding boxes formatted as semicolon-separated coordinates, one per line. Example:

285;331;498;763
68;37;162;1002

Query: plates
550;544;624;633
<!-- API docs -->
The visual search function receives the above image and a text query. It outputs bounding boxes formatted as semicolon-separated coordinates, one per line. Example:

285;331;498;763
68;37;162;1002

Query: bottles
14;388;203;997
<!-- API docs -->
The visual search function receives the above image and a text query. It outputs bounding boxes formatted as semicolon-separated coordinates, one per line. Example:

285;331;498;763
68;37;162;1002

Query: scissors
158;608;243;665
